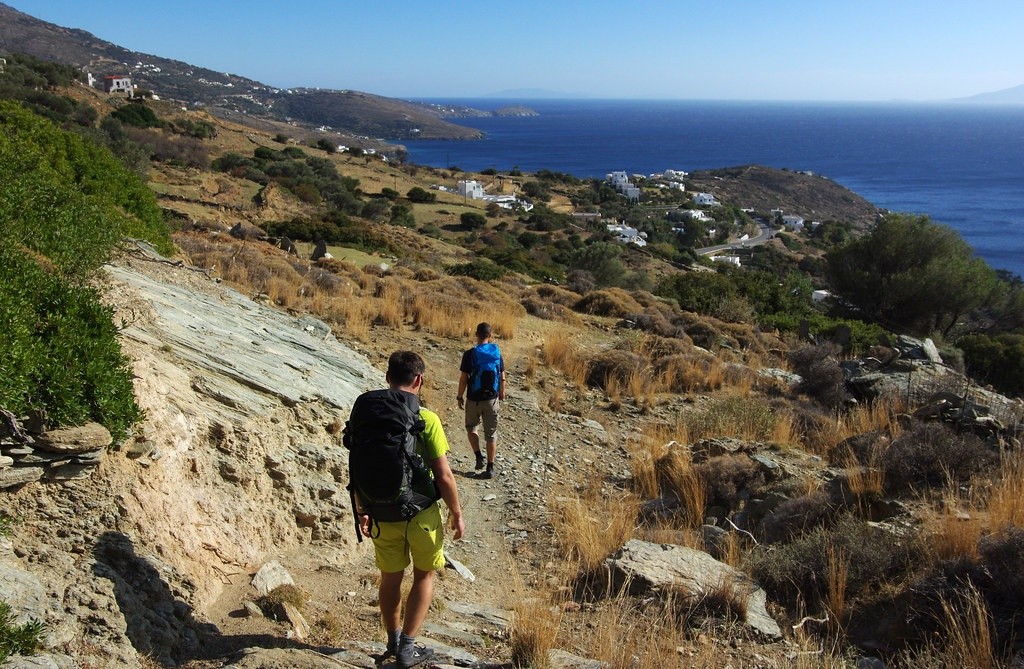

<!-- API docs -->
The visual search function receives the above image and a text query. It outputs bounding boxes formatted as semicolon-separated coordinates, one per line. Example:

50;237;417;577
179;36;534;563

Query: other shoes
475;459;485;470
486;467;494;478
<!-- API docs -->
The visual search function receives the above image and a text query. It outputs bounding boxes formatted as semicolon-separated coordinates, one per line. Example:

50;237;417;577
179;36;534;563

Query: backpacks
343;389;441;523
467;342;501;398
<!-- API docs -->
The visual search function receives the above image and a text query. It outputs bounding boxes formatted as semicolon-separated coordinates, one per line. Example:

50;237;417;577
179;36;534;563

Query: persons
355;350;464;669
457;322;505;477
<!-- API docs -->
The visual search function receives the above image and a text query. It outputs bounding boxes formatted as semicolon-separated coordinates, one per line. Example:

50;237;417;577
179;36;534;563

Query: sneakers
387;636;400;654
395;642;434;669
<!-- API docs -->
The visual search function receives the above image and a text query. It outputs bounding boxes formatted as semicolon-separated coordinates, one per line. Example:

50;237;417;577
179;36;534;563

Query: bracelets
457;396;462;400
357;512;366;516
